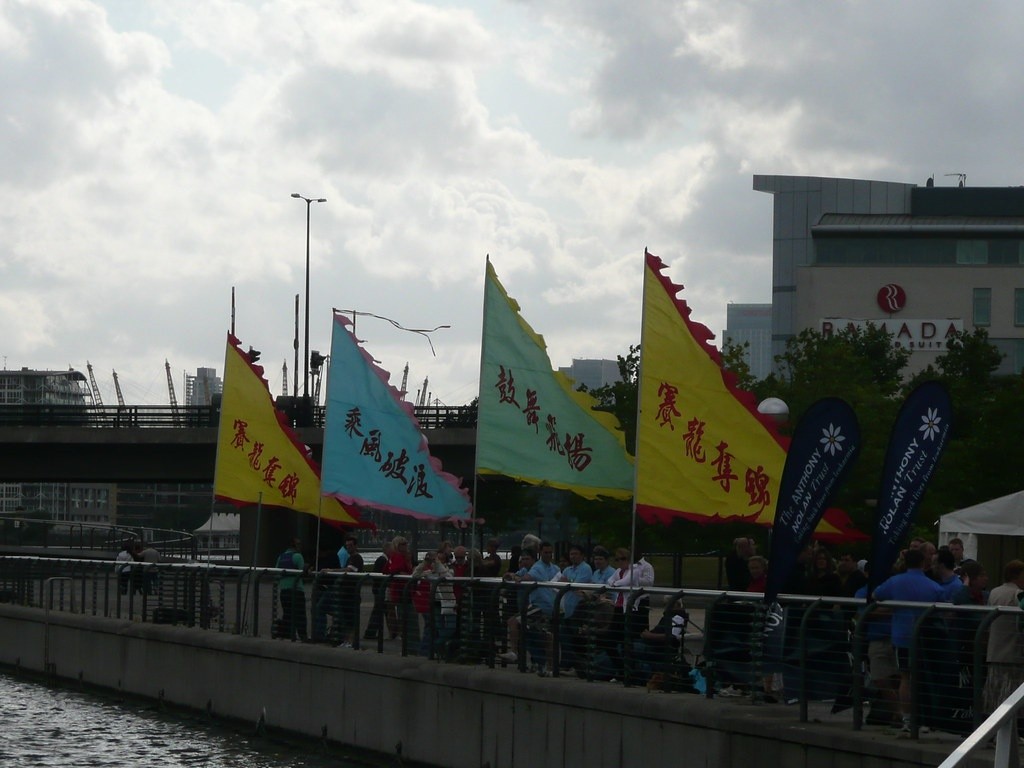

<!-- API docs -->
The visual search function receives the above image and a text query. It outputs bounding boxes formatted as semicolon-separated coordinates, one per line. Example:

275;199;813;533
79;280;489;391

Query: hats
856;559;868;573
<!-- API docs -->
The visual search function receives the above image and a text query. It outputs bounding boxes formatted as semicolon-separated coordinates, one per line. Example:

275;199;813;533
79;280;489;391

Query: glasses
615;557;627;562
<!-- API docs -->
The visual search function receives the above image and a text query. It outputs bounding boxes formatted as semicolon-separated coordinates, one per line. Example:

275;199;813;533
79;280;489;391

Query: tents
192;513;241;548
938;492;1024;563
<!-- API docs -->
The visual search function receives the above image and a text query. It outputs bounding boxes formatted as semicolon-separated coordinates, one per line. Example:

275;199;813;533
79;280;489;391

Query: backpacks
278;549;299;569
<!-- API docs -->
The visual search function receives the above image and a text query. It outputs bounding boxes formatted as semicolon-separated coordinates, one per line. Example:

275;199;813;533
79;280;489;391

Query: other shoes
902;719;912;732
719;684;753;698
498;651;518;661
345;642;353;648
363;635;377;639
337;641;346;647
610;678;617;682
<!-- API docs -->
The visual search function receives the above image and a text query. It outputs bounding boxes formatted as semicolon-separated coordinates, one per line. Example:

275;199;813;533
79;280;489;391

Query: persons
383;536;413;637
274;538;309;642
321;537;363;648
364;542;398;639
502;533;690;684
413;538;502;669
703;537;1024;748
115;543;160;595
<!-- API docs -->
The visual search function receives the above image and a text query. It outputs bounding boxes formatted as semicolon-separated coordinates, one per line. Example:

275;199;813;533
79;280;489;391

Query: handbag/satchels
437;584;457;616
383;587;393;606
412;580;432;611
